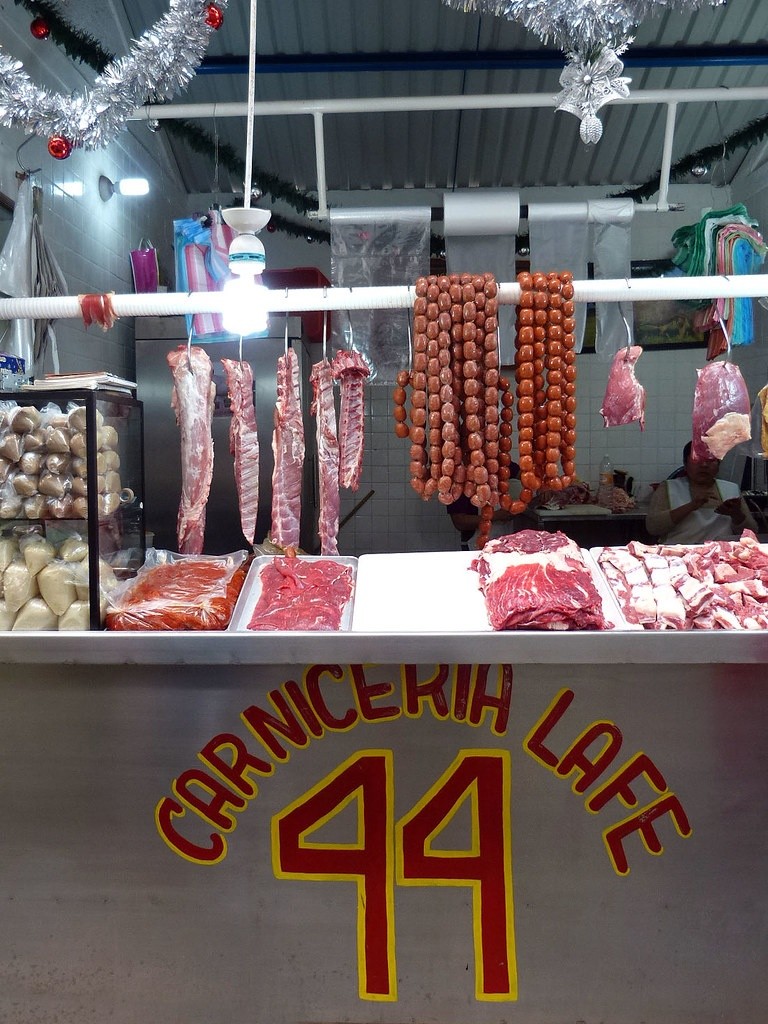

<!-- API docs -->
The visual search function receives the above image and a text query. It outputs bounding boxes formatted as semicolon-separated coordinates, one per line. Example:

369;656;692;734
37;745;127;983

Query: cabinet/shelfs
0;389;146;631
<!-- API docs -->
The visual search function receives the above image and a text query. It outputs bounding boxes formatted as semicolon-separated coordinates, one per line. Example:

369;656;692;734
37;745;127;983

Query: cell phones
714;496;739;513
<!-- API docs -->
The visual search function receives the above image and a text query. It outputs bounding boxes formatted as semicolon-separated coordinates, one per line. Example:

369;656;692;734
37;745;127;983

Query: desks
514;498;651;537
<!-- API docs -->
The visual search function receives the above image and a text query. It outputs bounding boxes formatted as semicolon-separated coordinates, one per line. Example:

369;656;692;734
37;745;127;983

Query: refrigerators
133;317;314;558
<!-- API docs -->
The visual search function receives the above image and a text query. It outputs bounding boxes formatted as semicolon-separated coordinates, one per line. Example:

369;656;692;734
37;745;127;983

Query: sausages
395;270;575;549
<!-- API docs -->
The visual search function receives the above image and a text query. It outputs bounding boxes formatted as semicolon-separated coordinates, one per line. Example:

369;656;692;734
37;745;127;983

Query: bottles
598;454;614;505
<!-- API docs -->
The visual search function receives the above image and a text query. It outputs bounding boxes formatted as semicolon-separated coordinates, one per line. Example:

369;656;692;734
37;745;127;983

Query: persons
646;440;759;546
447;462;527;551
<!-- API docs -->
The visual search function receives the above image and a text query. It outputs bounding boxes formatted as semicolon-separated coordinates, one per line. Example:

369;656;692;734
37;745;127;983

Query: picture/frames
575;258;711;355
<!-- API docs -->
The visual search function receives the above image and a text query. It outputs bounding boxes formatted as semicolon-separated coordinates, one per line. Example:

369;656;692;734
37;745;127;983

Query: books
21;371;138;395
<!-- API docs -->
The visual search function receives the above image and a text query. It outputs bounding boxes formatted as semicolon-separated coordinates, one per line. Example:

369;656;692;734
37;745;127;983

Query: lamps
216;0;271;335
